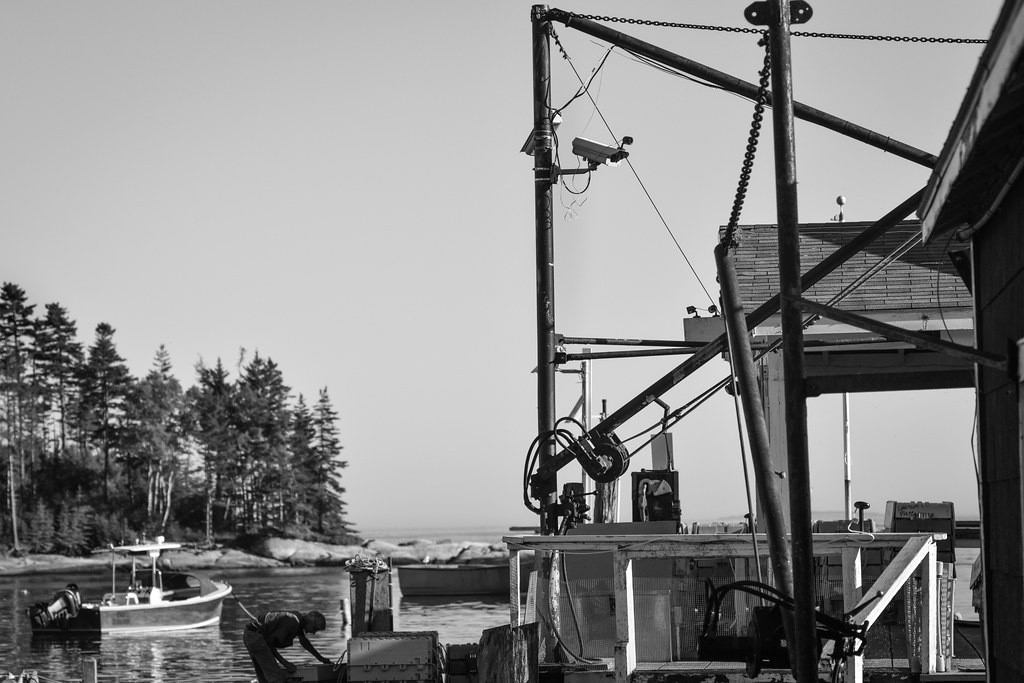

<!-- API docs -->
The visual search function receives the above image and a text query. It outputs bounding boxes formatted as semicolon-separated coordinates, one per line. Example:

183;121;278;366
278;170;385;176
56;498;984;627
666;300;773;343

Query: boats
25;534;233;634
397;551;541;600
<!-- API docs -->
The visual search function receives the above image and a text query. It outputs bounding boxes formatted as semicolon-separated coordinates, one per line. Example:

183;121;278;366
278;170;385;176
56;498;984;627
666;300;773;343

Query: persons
243;611;332;683
